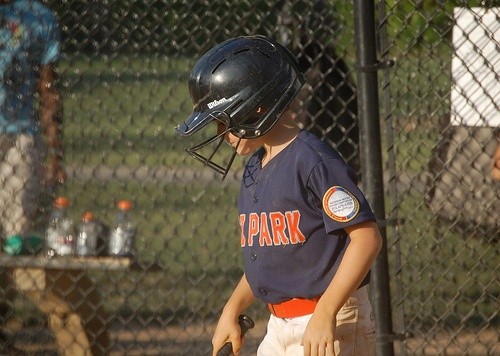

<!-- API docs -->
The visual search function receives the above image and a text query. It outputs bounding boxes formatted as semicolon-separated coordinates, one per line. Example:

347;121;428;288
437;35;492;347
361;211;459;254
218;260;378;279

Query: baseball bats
214;314;255;356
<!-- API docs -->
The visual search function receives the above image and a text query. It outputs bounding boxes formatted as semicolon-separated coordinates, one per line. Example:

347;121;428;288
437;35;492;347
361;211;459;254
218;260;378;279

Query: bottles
76;211;97;257
58;221;73;255
108;201;134;257
46;198;74;259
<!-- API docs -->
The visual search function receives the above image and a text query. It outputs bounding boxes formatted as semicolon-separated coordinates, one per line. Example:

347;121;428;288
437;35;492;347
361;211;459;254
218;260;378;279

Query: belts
268;269;370;318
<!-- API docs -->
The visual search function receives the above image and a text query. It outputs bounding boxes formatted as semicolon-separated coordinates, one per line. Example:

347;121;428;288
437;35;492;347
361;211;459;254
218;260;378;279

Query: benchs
0;255;164;356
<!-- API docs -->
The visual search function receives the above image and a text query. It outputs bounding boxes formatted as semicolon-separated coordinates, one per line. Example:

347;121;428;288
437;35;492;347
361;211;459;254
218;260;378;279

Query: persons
0;0;64;333
177;36;382;356
278;0;361;192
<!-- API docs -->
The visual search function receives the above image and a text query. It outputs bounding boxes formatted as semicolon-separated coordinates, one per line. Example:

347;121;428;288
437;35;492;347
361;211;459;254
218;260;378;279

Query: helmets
174;35;306;140
